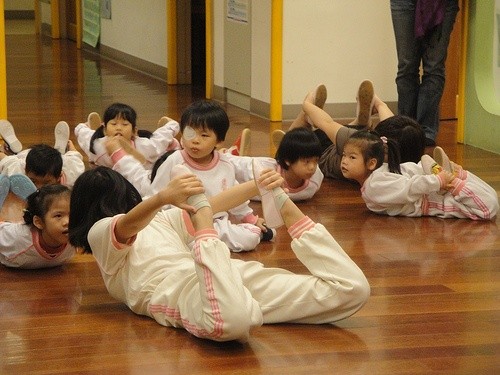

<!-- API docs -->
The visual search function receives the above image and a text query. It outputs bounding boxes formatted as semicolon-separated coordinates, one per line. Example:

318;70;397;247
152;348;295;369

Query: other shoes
421;154;448;195
0;175;10;210
305;84;328;129
230;128;253;156
170;164;208;215
0;119;23;154
251;156;284;228
53;121;70;156
88;112;102;130
260;222;277;242
157;116;168;130
433;147;451;175
272;129;286;148
9;174;37;201
354;79;375;127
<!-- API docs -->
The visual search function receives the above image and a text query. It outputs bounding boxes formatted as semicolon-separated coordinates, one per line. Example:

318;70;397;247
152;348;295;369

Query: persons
0;80;500;269
391;0;460;145
68;159;372;343
